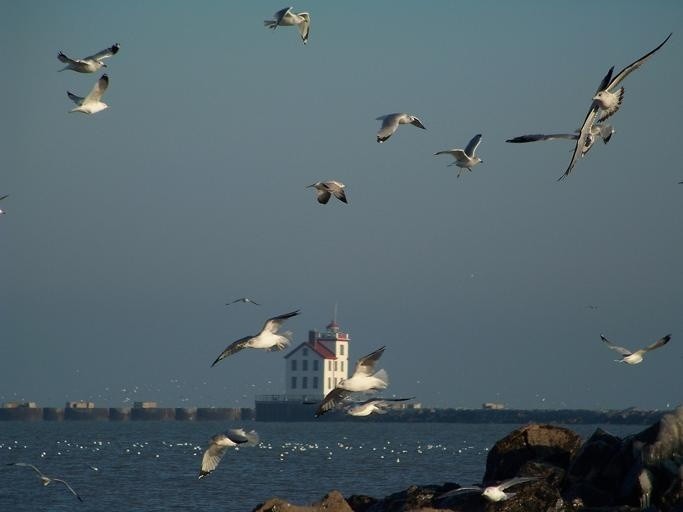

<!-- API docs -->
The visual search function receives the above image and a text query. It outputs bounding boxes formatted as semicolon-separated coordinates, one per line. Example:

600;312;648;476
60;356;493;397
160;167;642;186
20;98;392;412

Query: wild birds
207;296;302;370
596;333;672;367
302;178;351;211
312;344;417;418
197;424;248;480
260;9;312;45
55;42;121;117
4;459;85;506
504;31;672;181
431;131;488;176
373;110;427;144
435;474;542;503
631;438;654;511
